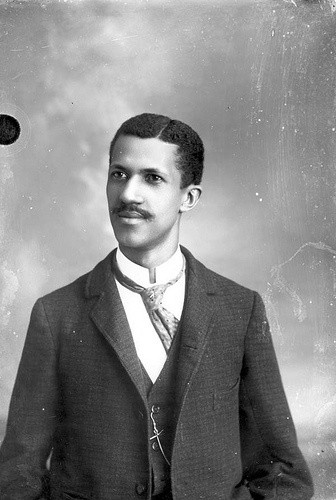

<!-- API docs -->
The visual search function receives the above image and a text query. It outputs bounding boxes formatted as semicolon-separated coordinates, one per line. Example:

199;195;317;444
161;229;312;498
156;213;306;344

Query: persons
1;112;314;499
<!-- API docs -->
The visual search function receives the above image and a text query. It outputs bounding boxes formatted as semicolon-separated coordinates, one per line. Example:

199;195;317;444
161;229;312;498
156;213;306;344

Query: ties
107;257;185;353
